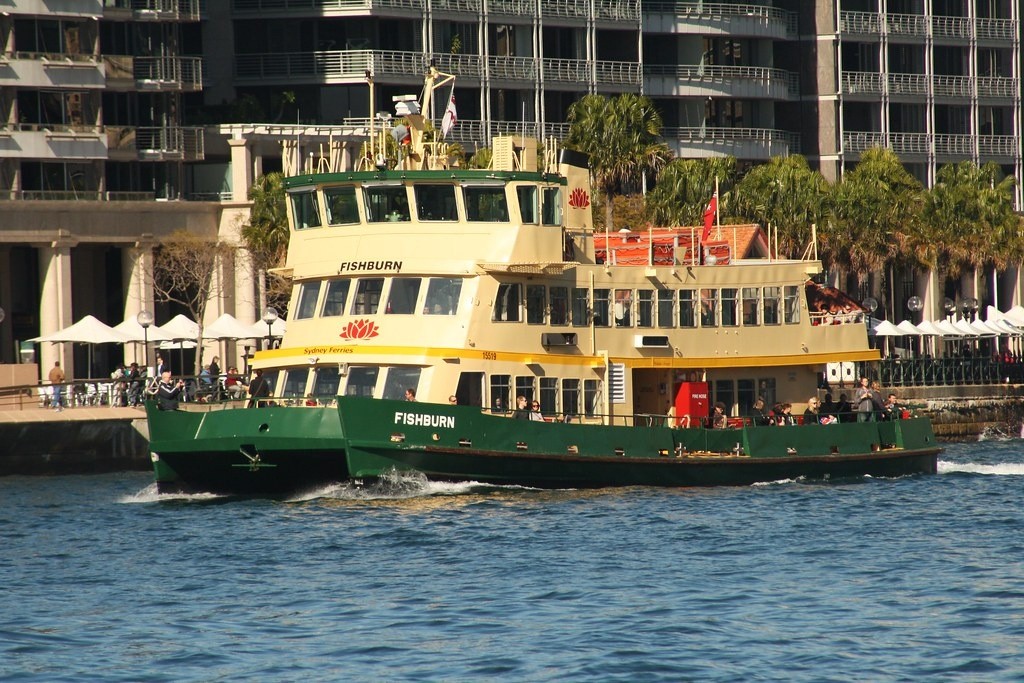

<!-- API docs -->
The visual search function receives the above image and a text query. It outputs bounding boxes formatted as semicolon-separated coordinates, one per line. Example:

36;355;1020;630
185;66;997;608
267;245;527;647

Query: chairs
36;372;248;410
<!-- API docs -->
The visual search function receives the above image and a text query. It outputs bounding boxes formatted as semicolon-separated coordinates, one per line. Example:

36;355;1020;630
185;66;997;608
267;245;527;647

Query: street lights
939;295;978;356
862;297;877;330
907;296;924;359
138;309;154;378
262;306;278;350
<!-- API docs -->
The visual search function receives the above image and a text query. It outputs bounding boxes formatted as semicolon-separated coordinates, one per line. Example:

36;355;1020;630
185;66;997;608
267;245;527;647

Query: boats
144;50;947;494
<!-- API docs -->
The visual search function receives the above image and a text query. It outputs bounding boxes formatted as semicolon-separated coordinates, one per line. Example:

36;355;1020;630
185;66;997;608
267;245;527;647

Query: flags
701;189;717;244
441;93;458;141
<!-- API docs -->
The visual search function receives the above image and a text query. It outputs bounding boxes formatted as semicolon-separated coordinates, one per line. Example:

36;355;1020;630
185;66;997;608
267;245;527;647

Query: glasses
533;405;539;408
520;399;527;402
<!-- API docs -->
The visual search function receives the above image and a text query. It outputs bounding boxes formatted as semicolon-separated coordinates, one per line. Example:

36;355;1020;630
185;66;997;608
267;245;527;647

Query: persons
423;306;430;314
750;394;774;424
835;393;853;422
528;401;545;421
448;395;458;404
772;402;797;426
888;393;907;414
708;403;739;428
822;393;836;414
881;397;895;419
866;378;890;420
402;389;419;402
267;391;278;408
306;392;319;406
494;398;505;409
510;396;531;419
854;376;875;421
870;343;1024;385
109;356;248;411
801;399;819;423
834;302;862;325
812;397;825;424
249;368;270;409
47;360;68;410
815;305;837;329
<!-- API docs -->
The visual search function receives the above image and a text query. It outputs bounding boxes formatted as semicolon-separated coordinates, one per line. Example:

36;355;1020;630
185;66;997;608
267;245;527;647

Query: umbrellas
866;300;1023;340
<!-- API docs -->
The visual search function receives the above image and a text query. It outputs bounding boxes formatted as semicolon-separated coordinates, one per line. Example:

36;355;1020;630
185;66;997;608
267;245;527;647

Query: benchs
792;415;805;425
727;418;751;429
